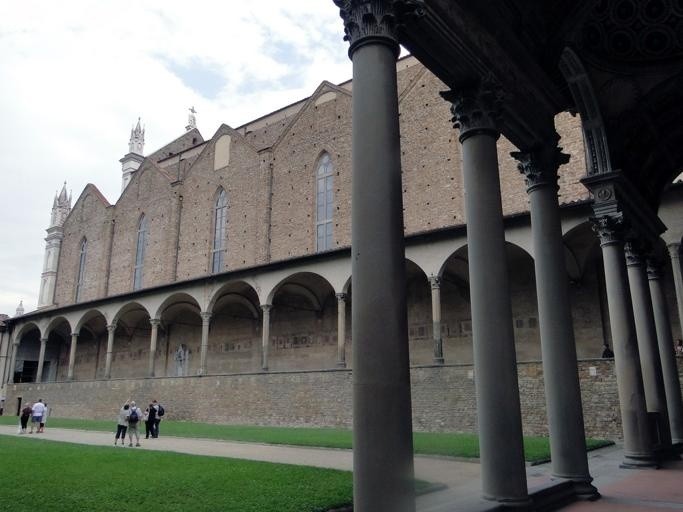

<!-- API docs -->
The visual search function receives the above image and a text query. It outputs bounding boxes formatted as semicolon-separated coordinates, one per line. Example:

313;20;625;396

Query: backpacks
130;410;137;423
157;405;164;416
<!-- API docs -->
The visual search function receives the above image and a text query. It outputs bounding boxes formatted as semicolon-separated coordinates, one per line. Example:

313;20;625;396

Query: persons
151;399;161;438
113;398;130;447
145;404;157;440
38;403;48;433
144;408;149;421
20;404;31;433
128;401;143;448
30;398;45;433
